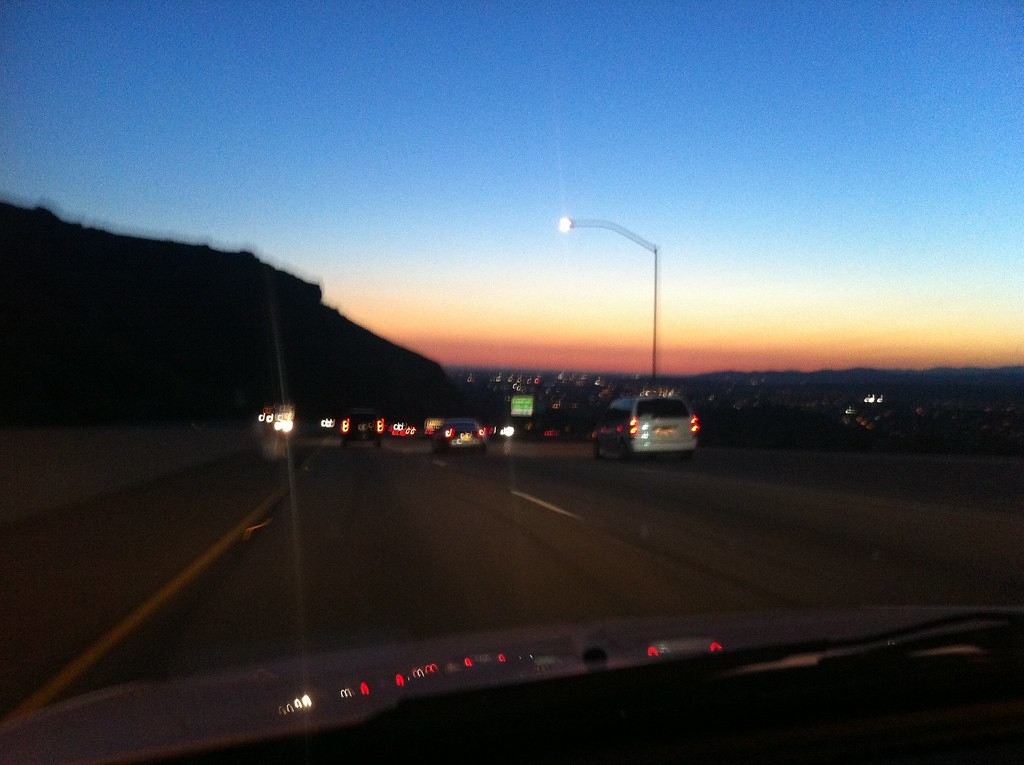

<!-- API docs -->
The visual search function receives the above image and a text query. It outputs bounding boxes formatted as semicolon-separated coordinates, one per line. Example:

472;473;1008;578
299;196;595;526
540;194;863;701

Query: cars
431;419;488;457
340;411;385;448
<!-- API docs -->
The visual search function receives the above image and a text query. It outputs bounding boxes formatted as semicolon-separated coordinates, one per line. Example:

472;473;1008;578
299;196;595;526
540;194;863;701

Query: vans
591;394;699;464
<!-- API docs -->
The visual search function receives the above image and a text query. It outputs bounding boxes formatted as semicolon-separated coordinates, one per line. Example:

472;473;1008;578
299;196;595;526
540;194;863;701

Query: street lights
556;216;658;385
